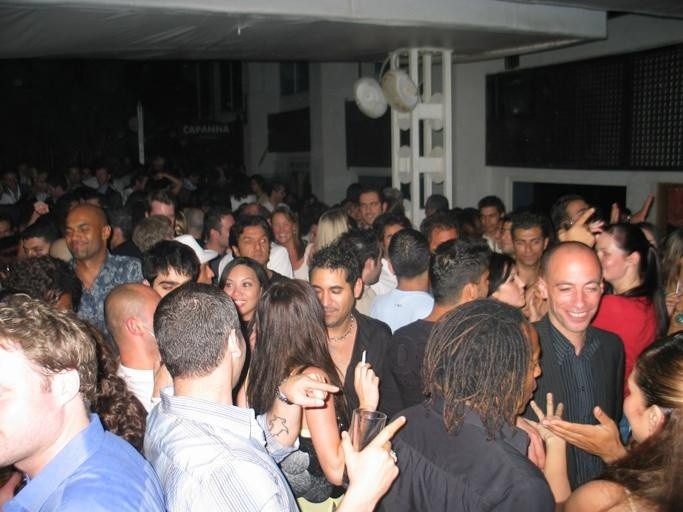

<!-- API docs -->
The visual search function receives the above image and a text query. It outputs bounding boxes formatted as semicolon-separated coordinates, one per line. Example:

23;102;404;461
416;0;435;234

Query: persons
1;149;682;512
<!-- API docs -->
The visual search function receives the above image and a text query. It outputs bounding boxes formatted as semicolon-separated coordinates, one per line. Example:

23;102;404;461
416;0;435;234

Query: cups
349;406;388;453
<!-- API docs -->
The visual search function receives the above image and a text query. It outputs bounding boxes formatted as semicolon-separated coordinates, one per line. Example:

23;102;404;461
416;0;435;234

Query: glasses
237;238;270;246
360;202;383;209
